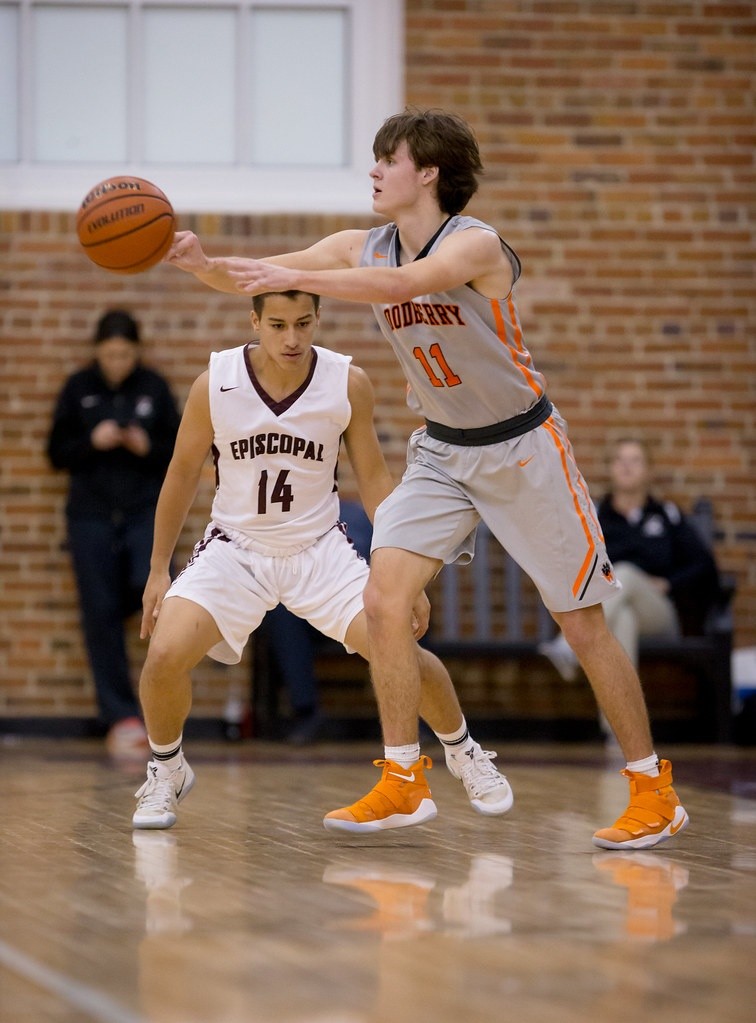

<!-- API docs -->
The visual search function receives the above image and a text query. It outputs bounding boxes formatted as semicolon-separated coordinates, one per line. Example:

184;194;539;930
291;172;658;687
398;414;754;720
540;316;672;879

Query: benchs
240;520;735;735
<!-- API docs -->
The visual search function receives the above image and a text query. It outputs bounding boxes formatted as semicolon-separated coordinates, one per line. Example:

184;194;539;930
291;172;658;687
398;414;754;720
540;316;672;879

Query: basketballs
75;174;177;277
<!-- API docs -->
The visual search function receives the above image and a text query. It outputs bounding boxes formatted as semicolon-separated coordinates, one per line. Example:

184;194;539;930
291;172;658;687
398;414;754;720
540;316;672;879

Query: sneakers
131;750;194;831
593;760;690;850
446;740;513;816
323;755;438;834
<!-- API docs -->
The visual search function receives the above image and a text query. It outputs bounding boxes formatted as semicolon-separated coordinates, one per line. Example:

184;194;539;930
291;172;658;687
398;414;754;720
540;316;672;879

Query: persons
41;306;373;762
160;112;689;850
539;433;729;762
132;289;513;827
